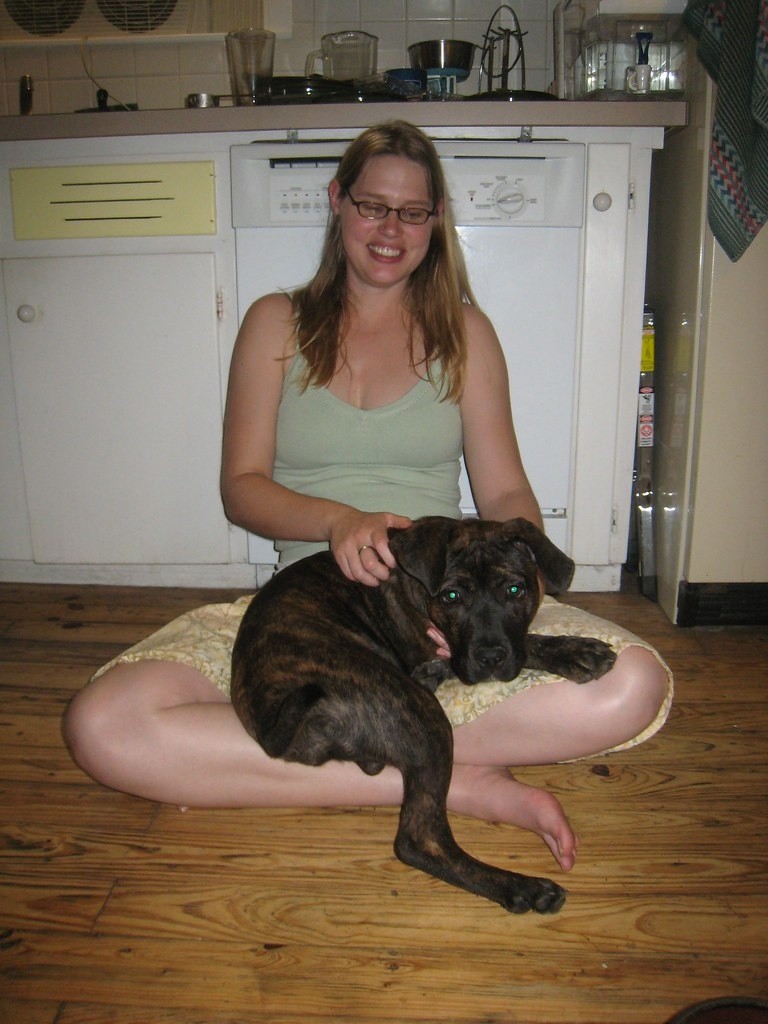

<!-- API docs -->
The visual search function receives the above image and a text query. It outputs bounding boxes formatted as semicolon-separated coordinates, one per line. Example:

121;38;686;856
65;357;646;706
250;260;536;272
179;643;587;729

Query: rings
358;545;376;555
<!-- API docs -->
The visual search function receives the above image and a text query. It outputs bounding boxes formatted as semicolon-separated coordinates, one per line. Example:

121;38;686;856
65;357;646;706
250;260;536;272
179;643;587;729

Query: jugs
304;31;379;83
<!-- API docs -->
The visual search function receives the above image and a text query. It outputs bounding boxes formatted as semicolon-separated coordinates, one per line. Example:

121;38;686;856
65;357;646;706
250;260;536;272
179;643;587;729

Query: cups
227;27;275;106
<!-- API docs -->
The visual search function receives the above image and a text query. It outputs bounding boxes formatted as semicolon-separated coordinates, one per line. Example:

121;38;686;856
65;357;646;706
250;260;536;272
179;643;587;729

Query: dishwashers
227;141;587;589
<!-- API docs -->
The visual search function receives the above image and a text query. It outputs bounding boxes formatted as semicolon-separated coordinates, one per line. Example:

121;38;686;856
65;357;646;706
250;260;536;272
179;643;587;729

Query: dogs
230;515;618;914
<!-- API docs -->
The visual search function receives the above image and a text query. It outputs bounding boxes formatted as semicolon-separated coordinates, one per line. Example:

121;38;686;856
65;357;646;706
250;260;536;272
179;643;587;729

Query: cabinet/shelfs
0;133;257;588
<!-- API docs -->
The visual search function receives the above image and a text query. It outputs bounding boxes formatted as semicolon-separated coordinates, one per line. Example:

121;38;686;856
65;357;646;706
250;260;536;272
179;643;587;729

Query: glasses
342;186;436;225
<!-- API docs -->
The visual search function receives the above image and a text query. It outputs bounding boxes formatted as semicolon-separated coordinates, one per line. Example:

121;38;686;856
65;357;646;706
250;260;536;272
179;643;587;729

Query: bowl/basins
406;38;477;83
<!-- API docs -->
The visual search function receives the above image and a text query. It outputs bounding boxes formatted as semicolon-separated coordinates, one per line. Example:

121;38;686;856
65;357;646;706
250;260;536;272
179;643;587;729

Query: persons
62;119;675;870
